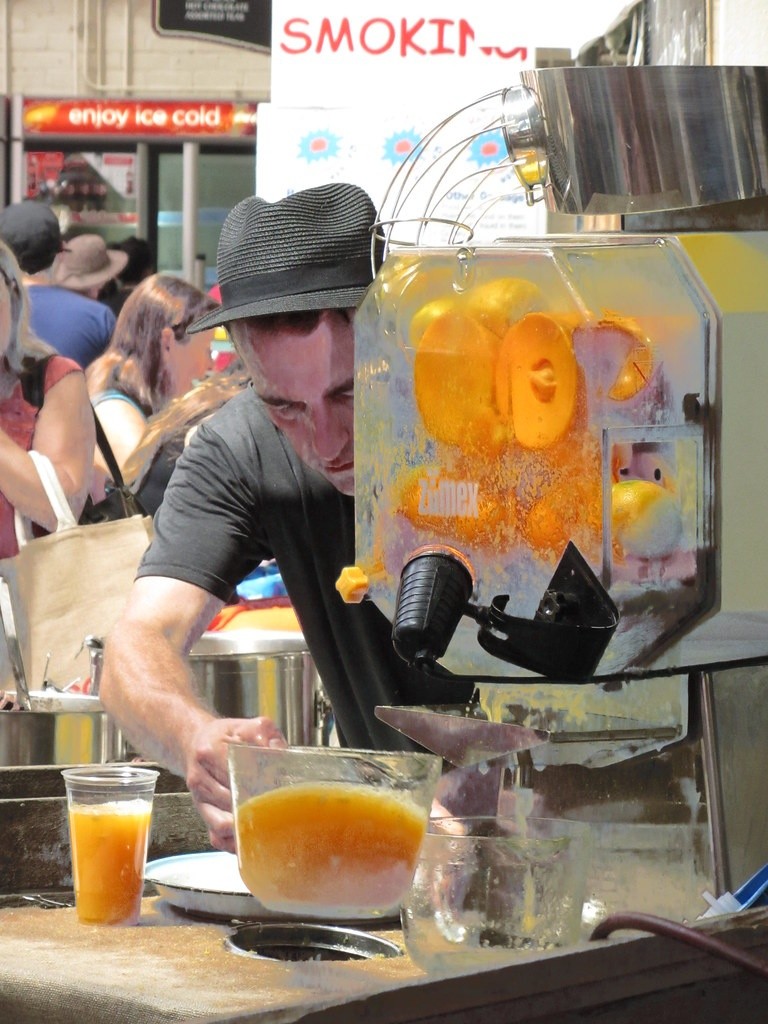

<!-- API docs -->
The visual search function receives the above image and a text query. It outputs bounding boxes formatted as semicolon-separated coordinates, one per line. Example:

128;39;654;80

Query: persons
0;244;97;691
98;181;515;853
46;232;156;319
1;205;119;368
83;271;224;505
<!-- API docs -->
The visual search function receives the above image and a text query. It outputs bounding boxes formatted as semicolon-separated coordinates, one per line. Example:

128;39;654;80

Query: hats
1;200;64;275
184;183;390;336
54;233;129;287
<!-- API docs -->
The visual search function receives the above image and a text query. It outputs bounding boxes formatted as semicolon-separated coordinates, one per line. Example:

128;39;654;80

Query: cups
60;767;164;927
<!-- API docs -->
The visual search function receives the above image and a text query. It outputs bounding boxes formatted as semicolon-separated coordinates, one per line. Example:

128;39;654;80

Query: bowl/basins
404;815;592;982
0;694;127;767
228;742;444;921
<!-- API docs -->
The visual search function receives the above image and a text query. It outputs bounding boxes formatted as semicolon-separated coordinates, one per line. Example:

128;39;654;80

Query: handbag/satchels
0;447;160;700
17;350;145;543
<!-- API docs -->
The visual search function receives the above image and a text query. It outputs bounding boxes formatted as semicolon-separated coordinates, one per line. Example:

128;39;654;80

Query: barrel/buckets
184;646;333;750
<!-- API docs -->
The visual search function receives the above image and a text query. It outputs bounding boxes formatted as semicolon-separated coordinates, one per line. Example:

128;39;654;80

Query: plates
145;850;299;921
